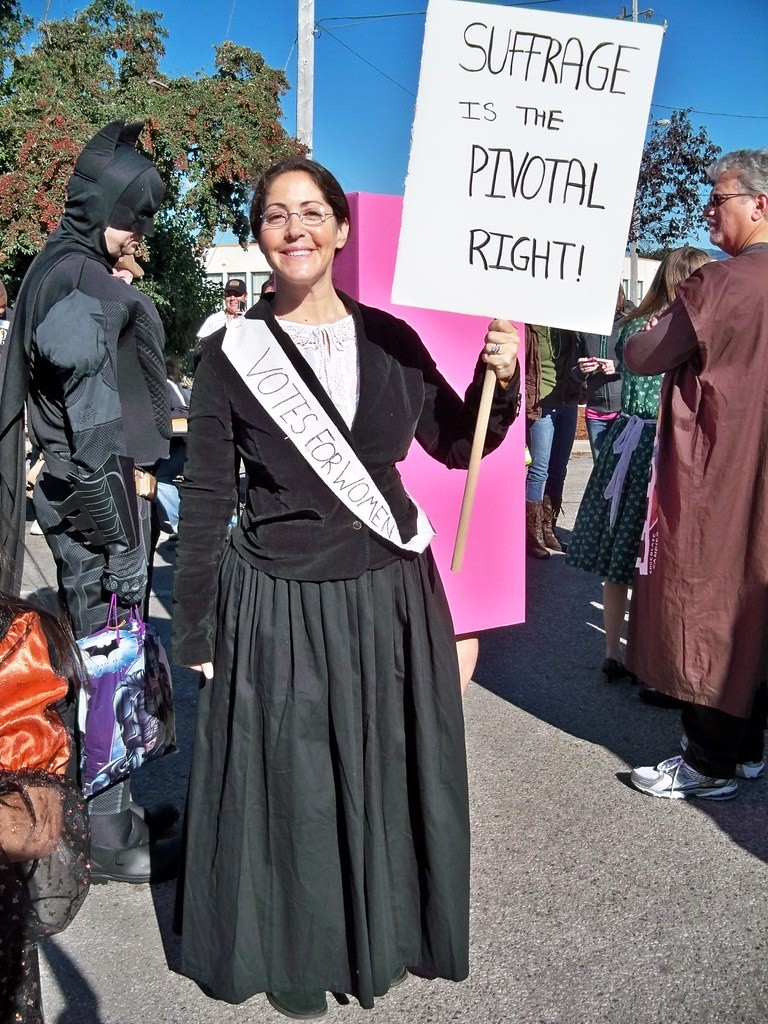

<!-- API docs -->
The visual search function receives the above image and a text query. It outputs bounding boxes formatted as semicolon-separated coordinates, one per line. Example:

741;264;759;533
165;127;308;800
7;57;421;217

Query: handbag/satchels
74;595;181;800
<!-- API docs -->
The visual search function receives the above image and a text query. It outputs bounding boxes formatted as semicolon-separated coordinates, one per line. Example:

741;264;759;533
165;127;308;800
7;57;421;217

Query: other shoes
639;687;682;706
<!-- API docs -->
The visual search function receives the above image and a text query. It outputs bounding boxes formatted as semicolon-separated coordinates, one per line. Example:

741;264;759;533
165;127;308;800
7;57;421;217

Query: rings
492;344;500;354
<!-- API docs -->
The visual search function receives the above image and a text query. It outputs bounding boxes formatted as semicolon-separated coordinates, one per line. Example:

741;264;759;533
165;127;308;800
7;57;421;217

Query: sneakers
630;753;739;802
680;730;766;779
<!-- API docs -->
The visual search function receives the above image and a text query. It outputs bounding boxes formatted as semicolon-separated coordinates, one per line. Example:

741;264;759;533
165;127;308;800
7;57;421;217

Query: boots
542;495;567;551
526;499;550;559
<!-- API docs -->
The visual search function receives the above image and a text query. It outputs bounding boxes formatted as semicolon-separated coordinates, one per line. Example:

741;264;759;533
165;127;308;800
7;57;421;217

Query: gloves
103;548;148;606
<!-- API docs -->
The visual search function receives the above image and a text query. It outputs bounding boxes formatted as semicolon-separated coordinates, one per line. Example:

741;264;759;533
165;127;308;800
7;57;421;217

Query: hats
225;279;246;293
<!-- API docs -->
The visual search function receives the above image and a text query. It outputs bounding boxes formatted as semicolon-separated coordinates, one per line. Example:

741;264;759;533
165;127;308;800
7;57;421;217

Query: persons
0;591;91;1024
524;324;588;559
196;278;248;338
566;247;710;684
570;282;636;463
0;119;185;885
170;158;522;1015
624;149;768;798
0;281;12;346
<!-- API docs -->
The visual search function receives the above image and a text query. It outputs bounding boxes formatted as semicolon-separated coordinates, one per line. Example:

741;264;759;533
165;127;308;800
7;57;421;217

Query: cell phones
238;300;245;316
587;357;606;371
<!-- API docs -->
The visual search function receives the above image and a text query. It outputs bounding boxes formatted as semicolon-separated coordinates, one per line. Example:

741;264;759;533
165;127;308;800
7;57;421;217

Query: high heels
603;657;628;683
629;670;639;686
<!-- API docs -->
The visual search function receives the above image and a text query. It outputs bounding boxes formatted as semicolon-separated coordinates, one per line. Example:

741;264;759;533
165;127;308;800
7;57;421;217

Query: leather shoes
127;803;181;831
87;841;189;886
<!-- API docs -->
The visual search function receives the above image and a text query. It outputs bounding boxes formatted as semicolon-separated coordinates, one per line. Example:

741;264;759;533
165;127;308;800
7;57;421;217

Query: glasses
709;194;759;207
258;208;337;228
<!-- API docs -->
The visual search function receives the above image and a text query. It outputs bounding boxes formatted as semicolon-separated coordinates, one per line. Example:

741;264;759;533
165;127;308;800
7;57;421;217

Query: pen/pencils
572;357;596;369
599;362;604;365
